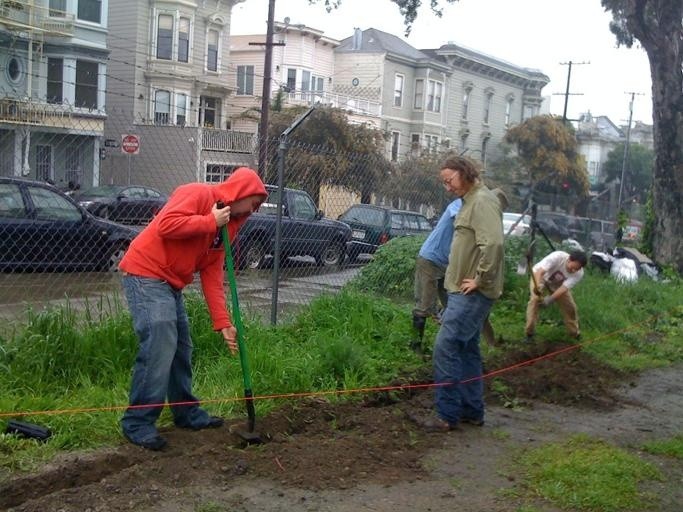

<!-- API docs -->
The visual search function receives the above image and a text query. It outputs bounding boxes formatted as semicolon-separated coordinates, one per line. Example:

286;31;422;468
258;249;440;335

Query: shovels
216;200;268;444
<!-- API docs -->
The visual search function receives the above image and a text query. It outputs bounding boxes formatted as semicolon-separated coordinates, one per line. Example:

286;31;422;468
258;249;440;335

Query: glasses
443;171;458;187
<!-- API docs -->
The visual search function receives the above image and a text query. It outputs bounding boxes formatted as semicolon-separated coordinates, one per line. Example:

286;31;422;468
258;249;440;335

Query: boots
409;315;426;355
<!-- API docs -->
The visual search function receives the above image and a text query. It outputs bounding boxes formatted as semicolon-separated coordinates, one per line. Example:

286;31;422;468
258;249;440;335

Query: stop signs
122;134;142;154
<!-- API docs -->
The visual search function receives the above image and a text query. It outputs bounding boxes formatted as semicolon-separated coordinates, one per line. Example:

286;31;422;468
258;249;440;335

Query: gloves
534;282;554;308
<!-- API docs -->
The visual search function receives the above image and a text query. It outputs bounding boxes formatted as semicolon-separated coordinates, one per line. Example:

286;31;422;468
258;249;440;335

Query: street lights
269;107;320;333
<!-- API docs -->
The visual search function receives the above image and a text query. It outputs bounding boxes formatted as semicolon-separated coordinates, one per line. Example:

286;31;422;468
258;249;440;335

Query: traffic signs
105;138;121;149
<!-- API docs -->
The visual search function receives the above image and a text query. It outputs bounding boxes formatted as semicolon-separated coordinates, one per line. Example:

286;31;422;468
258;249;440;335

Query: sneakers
521;336;535;347
417;416;454;433
202;412;224;429
122;429;168;449
463;414;485;427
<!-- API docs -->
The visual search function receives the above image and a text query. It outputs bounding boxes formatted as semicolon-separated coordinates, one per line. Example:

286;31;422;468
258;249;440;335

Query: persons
410;155;510;432
525;249;587;344
117;167;268;451
412;187;510;361
